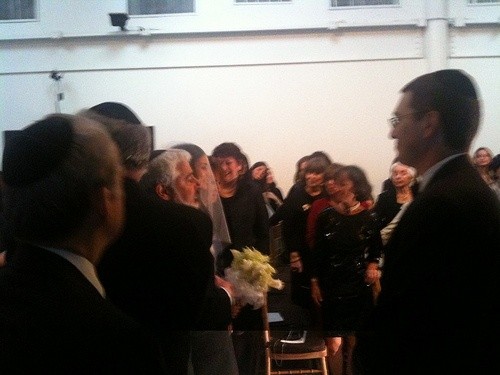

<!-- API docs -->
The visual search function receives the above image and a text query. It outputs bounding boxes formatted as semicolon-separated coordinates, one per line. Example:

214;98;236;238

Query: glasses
390;109;436;128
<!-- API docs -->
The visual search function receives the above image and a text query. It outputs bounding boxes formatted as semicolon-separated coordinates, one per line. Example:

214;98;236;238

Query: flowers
224;244;285;310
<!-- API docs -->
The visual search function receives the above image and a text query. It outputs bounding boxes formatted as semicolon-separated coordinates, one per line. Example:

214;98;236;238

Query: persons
143;140;500;375
0;114;124;375
350;68;500;375
60;100;233;375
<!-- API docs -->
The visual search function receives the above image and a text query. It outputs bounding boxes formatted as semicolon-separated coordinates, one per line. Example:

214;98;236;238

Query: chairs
259;288;330;375
